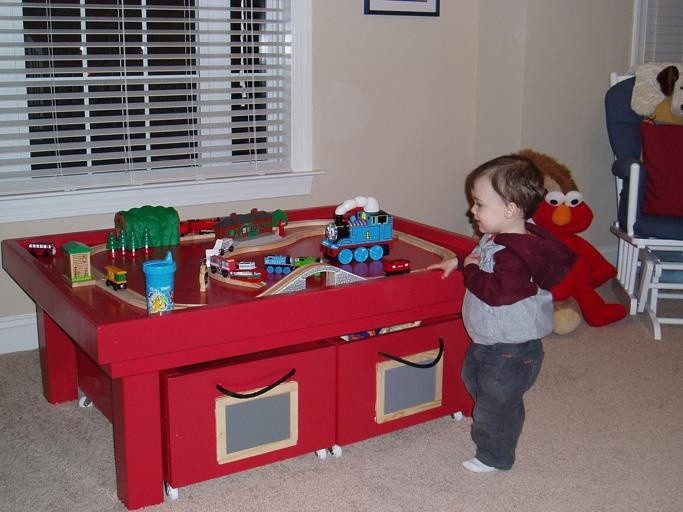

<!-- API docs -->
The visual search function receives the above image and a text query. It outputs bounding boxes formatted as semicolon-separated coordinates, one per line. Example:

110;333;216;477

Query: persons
426;156;577;474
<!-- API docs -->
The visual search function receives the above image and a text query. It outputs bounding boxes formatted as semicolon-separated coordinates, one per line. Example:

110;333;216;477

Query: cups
142;250;177;314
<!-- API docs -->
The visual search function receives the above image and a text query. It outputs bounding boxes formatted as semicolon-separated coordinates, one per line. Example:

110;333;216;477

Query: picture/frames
363;0;440;18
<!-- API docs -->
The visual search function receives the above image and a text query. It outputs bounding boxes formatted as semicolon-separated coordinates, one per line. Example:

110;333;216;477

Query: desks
1;205;479;510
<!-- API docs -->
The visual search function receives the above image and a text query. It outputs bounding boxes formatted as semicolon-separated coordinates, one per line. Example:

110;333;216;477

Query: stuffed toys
517;147;626;335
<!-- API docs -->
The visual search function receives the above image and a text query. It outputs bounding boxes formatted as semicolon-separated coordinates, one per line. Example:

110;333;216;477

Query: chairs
606;72;683;342
